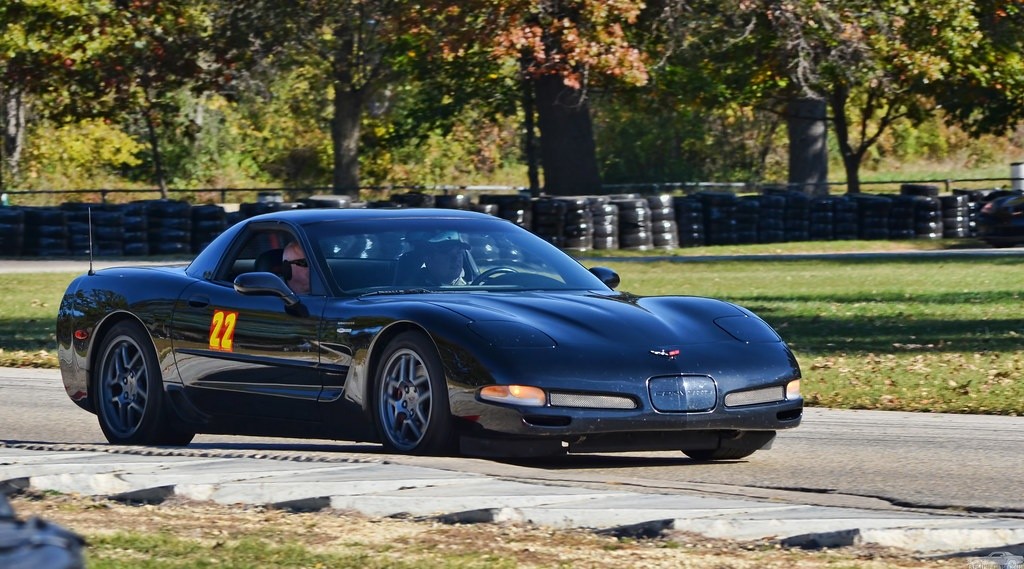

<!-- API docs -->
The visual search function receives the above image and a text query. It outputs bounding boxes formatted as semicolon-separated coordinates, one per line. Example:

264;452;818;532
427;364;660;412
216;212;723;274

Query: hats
425;230;471;250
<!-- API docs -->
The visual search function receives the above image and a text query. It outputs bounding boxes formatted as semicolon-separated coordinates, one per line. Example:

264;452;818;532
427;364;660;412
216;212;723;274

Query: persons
415;230;472;287
281;239;311;296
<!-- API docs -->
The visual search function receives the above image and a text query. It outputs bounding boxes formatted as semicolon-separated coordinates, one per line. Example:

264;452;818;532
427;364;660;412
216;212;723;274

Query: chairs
254;248;285;275
393;251;426;286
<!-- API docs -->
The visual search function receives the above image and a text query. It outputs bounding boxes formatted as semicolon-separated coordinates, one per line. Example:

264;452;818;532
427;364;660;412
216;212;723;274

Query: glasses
286;258;308;268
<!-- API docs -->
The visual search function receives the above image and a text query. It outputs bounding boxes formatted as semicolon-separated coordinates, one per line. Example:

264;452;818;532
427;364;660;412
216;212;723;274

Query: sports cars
56;211;804;461
975;194;1024;247
